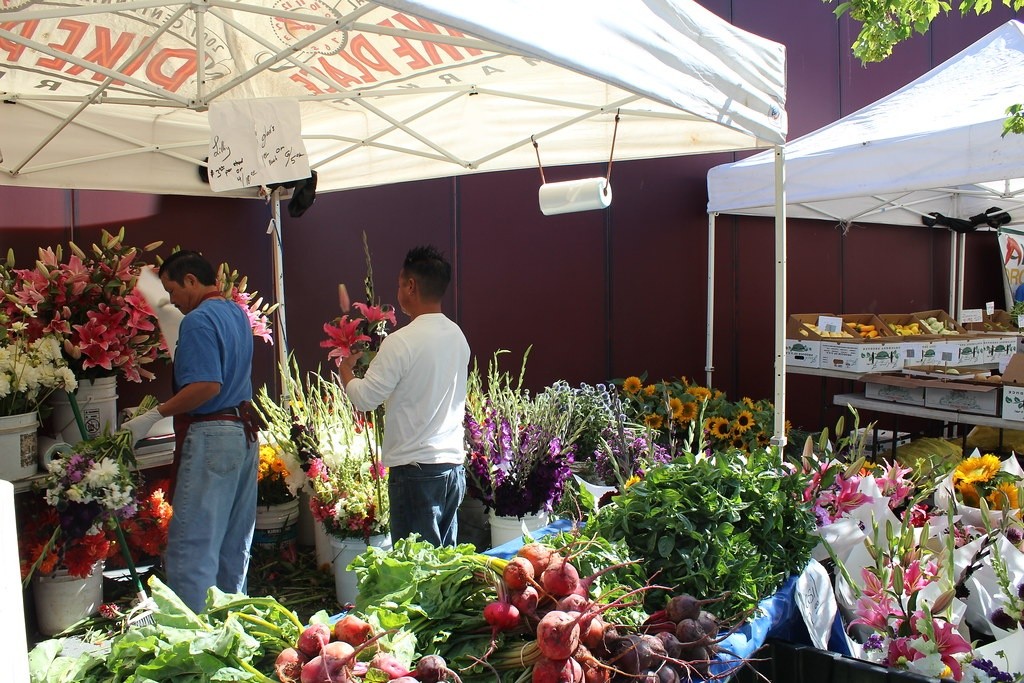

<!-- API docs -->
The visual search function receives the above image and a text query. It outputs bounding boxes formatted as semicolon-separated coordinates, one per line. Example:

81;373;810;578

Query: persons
338;243;471;554
120;247;261;620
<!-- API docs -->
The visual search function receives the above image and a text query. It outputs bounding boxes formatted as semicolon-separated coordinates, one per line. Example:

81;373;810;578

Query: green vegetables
590;443;814;620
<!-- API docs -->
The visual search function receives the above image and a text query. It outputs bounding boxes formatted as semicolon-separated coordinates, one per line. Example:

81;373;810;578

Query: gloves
121;406;165;450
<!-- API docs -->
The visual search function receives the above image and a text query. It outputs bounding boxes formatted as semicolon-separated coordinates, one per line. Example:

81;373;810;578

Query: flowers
0;227;797;574
801;401;1024;683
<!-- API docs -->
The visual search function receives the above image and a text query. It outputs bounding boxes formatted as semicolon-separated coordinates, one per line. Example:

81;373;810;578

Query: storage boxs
786;310;1024;418
849;428;911;459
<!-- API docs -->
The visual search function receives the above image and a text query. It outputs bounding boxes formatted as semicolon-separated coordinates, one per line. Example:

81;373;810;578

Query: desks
14;450;175;496
834;393;1024;474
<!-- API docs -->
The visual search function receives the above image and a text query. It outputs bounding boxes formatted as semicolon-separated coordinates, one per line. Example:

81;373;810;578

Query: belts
195;408;236;418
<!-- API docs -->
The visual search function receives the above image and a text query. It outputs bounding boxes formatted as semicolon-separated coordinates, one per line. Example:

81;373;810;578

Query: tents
704;15;1024;475
3;0;789;577
